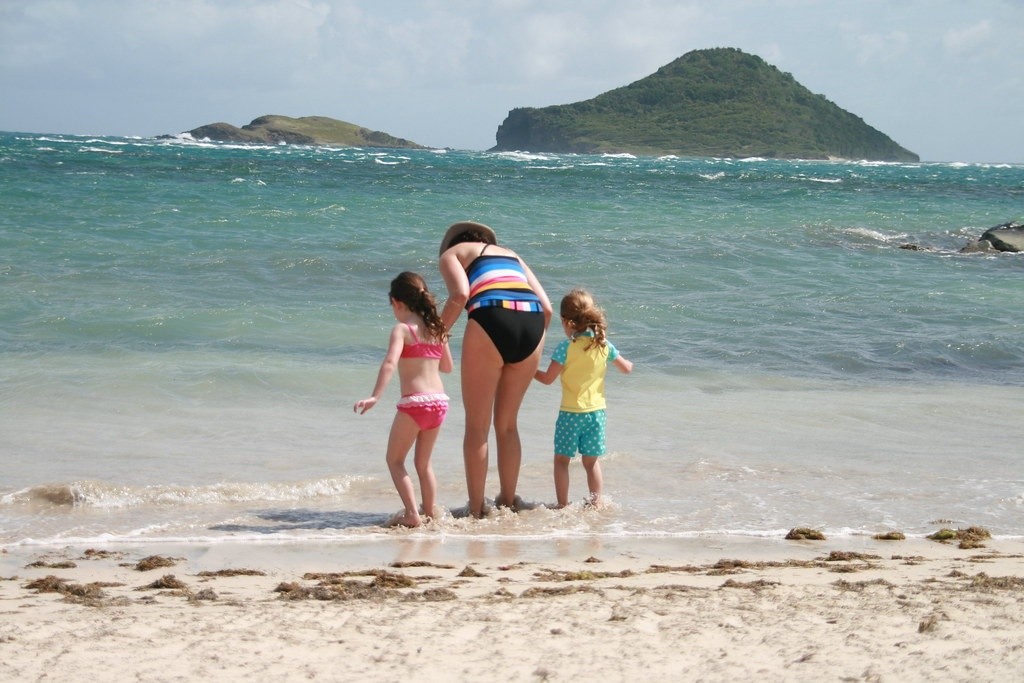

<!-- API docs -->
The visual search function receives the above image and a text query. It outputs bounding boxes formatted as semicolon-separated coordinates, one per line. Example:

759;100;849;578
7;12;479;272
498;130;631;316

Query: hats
440;221;497;256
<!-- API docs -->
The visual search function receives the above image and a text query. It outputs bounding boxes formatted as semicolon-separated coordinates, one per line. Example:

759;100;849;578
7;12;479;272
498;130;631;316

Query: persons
438;222;553;514
353;272;453;526
534;289;633;505
